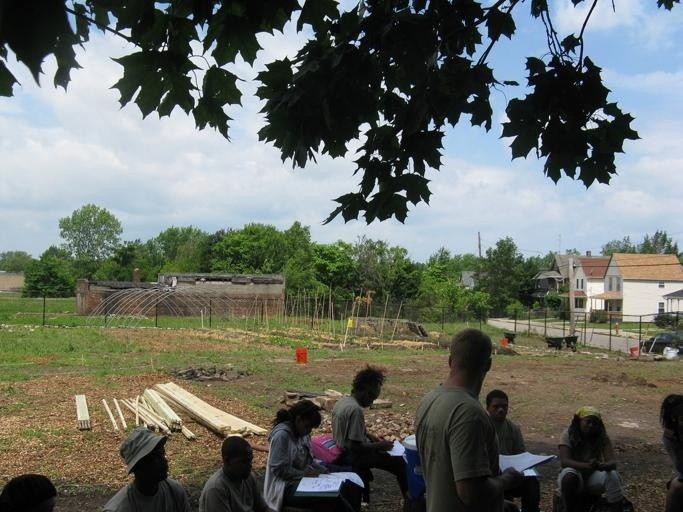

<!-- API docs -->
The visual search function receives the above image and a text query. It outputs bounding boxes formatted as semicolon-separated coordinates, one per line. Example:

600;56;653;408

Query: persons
102;426;192;512
0;474;58;512
331;364;409;501
198;436;276;512
556;405;624;511
263;399;362;512
485;389;540;512
414;327;524;512
660;393;683;512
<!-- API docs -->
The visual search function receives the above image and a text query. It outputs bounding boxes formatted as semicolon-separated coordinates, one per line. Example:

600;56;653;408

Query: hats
119;427;168;474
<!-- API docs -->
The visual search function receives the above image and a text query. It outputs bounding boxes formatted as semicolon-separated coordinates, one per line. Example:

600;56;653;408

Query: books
499;451;557;473
293;474;346;497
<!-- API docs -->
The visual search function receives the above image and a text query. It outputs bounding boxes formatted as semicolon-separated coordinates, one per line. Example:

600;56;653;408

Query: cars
640;332;683;355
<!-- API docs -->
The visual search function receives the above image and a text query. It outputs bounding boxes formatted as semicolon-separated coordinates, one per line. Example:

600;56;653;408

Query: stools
283;505;305;512
321;462;371;503
553;495;602;512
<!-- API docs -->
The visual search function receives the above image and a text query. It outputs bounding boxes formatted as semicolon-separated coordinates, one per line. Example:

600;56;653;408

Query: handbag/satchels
311;436;341;464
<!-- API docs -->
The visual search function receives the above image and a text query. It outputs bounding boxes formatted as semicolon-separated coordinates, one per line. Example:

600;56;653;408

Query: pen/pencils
378;435;387;441
306;464;314;470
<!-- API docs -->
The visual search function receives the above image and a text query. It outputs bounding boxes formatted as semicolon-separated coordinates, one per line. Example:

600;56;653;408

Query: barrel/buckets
295;348;307;362
401;434;427;499
630;345;639;356
500;338;508;345
